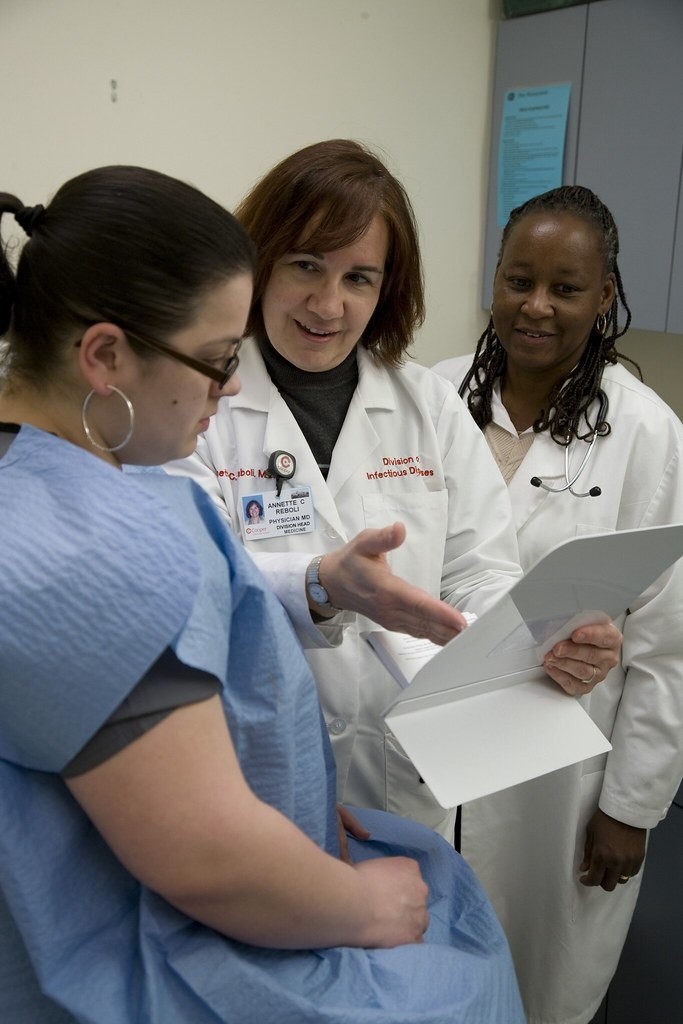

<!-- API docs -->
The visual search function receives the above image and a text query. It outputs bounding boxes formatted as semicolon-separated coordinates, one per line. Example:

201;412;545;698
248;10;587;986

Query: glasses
74;327;242;389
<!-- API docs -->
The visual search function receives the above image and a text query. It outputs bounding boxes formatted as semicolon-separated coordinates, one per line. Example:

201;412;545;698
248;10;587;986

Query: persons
161;137;621;837
436;181;683;1024
0;165;525;1024
246;500;264;525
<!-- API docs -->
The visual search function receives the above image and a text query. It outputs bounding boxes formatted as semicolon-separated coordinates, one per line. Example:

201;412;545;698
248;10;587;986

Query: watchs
307;555;341;612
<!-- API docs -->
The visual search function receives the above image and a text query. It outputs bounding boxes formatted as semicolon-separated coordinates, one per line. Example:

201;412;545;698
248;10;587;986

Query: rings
620;875;629;880
582;668;596;683
